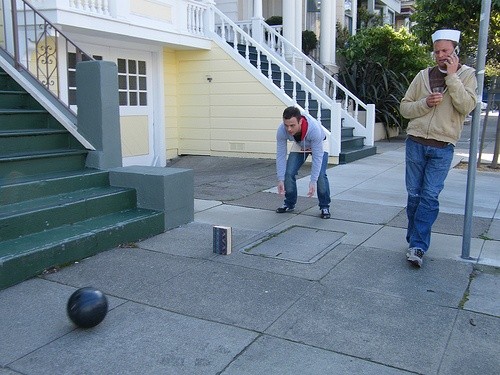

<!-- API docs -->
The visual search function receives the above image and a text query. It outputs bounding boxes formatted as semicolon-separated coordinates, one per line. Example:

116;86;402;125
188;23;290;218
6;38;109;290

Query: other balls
66;286;108;329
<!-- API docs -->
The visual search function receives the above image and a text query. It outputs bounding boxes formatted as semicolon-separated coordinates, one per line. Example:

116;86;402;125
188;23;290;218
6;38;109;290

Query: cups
433;87;443;95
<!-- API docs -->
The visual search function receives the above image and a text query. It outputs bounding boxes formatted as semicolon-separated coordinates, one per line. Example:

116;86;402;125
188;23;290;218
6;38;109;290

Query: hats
431;29;461;43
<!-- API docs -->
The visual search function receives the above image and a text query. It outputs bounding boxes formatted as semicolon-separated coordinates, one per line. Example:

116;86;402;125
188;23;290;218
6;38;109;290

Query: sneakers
406;246;424;268
275;202;295;213
321;208;330;219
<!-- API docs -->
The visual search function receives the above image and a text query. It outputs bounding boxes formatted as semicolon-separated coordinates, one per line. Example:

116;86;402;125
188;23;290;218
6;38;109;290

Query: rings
450;62;453;64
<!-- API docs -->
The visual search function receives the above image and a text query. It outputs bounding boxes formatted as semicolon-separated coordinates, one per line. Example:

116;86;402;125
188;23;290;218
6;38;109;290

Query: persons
399;29;478;268
277;106;331;219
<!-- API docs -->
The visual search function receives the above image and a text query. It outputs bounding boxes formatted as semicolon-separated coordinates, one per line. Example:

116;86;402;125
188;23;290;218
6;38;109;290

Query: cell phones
445;46;460;64
